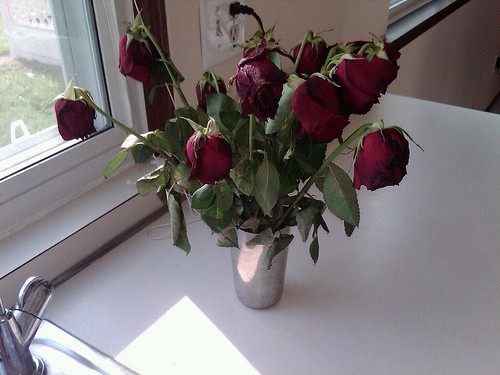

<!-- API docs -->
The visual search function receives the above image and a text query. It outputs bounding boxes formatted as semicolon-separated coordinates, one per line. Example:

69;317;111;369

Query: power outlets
199;1;246;71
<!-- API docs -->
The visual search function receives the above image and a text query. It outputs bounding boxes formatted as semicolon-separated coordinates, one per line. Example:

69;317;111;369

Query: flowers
53;0;427;271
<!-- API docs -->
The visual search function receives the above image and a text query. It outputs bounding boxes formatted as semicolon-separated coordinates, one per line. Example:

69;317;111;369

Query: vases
228;223;292;310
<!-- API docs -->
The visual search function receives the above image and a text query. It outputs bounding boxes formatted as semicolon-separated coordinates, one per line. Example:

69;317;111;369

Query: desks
34;92;499;373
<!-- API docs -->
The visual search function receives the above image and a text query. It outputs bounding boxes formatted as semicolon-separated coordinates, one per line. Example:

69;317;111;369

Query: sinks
27;318;143;375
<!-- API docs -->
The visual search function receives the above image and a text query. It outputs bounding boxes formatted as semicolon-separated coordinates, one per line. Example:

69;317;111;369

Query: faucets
0;276;56;375
